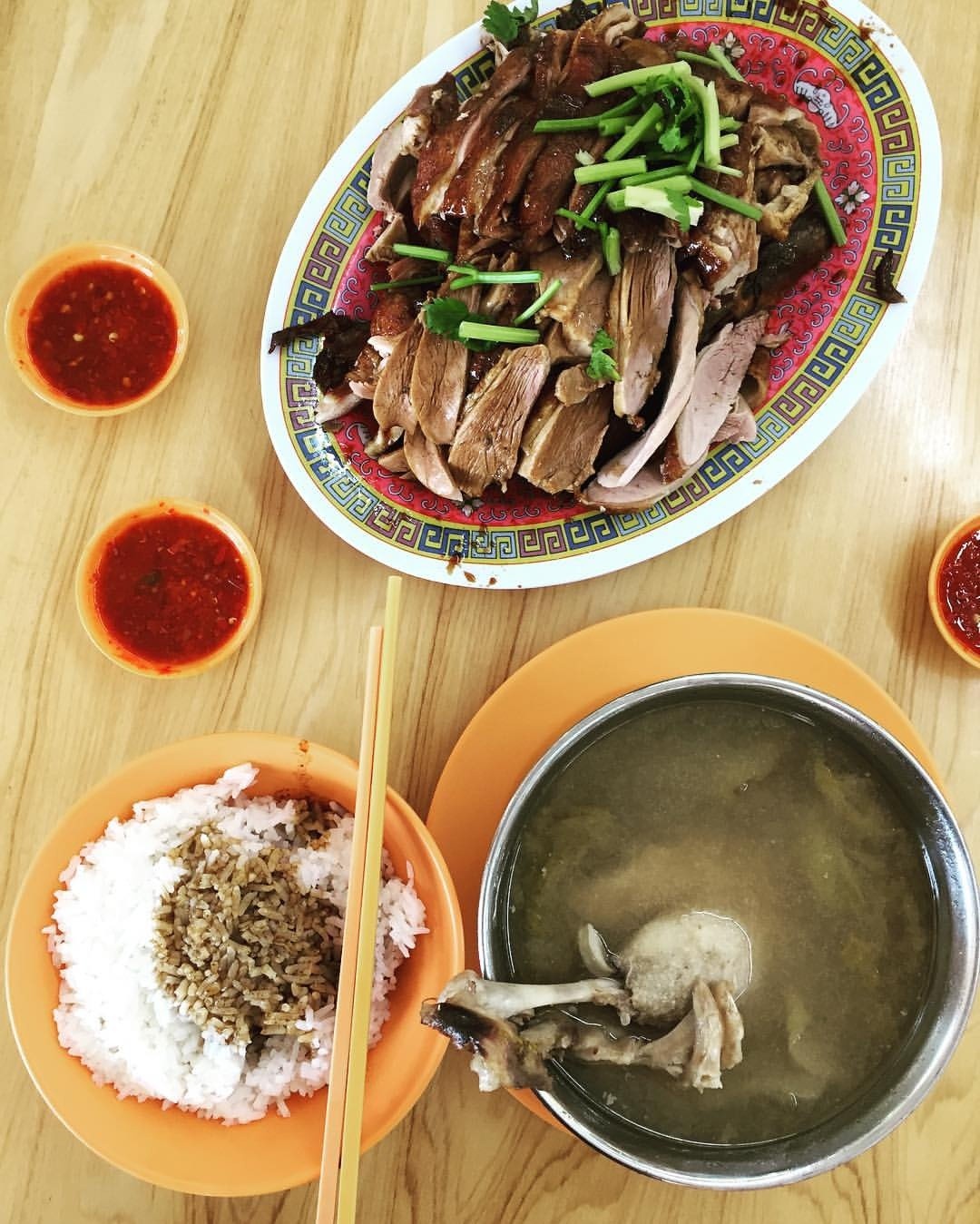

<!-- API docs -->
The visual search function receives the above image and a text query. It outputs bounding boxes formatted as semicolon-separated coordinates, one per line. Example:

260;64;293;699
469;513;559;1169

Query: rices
43;763;431;1132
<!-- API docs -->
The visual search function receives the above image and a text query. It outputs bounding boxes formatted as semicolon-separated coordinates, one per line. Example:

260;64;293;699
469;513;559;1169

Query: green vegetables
368;0;850;381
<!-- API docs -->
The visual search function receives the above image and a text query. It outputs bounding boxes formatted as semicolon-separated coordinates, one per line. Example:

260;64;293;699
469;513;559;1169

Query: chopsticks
315;576;403;1224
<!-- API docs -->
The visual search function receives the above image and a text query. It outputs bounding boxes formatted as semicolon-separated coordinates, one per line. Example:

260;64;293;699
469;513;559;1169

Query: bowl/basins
475;669;979;1194
4;732;466;1200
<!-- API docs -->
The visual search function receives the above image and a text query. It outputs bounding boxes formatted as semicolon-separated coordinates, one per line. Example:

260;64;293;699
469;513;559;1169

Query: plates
3;239;190;418
260;0;946;590
74;496;263;682
927;514;980;669
426;605;957;1138
